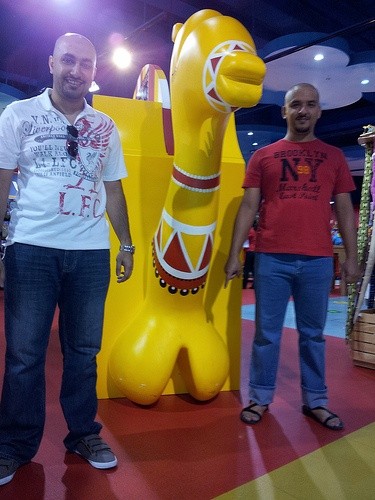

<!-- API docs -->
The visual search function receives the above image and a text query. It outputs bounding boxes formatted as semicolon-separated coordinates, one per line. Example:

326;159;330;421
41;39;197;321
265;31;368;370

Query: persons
0;33;135;486
225;82;361;429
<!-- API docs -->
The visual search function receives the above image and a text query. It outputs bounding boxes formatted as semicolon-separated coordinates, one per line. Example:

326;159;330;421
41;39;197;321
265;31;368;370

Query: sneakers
0;455;21;486
75;434;117;470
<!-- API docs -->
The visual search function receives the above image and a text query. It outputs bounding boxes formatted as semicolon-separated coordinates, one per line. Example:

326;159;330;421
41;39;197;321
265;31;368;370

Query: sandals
240;400;268;424
302;404;344;428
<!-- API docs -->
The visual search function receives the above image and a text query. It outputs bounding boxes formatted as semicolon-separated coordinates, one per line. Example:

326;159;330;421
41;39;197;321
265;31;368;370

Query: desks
333;246;346;296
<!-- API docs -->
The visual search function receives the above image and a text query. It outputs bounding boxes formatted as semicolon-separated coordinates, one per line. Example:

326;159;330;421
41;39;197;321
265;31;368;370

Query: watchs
119;244;135;255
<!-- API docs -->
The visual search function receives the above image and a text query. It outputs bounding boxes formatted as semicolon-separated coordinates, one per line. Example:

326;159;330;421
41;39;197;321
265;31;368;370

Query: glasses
65;124;78;159
